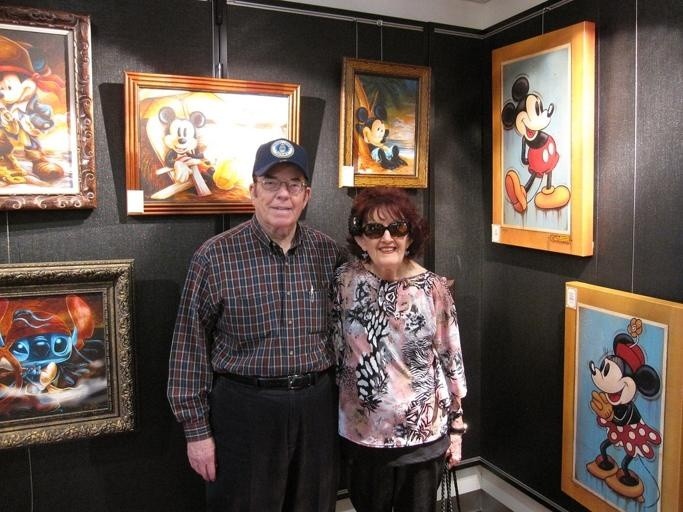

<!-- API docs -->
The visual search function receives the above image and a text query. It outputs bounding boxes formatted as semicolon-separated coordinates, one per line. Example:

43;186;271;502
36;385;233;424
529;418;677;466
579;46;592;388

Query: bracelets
447;422;469;435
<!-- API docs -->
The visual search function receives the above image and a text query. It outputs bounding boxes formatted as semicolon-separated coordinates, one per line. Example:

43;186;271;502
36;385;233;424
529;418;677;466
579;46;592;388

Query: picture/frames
492;21;595;257
338;57;432;189
0;6;96;209
561;281;683;512
124;72;301;216
0;259;140;450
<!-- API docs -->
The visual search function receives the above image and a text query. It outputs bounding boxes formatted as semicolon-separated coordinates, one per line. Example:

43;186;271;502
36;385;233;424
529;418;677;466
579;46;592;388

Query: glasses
359;221;412;240
252;179;309;193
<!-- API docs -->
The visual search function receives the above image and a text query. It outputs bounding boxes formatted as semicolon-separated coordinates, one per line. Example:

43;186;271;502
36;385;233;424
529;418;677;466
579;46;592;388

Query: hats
251;136;311;181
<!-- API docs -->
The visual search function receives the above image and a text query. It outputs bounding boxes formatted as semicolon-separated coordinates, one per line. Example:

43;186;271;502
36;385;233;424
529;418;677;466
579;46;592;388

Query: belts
216;365;330;390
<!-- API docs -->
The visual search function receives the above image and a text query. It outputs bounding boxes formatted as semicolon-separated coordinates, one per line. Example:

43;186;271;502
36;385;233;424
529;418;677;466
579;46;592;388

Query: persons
331;185;472;511
166;139;455;510
